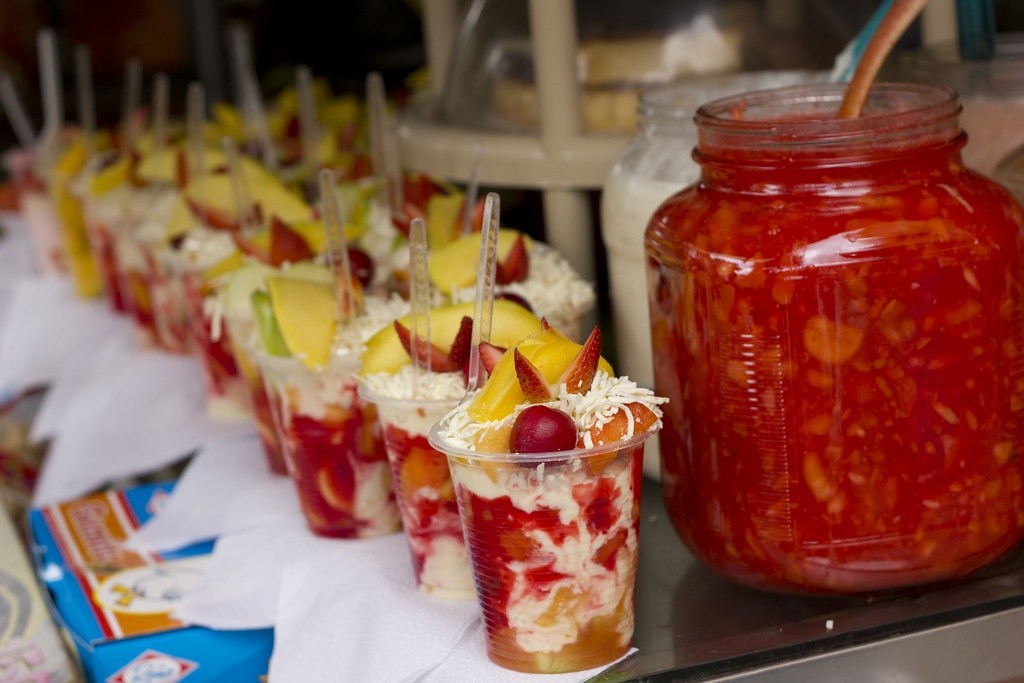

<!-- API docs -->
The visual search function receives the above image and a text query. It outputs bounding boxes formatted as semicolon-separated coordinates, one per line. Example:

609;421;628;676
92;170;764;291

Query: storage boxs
25;478;276;683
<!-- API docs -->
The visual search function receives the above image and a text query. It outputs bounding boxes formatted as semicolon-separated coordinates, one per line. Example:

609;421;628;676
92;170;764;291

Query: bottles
899;30;1024;201
602;67;838;485
643;81;1022;596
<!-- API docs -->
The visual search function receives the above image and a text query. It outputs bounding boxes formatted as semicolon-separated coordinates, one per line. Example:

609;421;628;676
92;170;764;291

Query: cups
0;111;658;676
